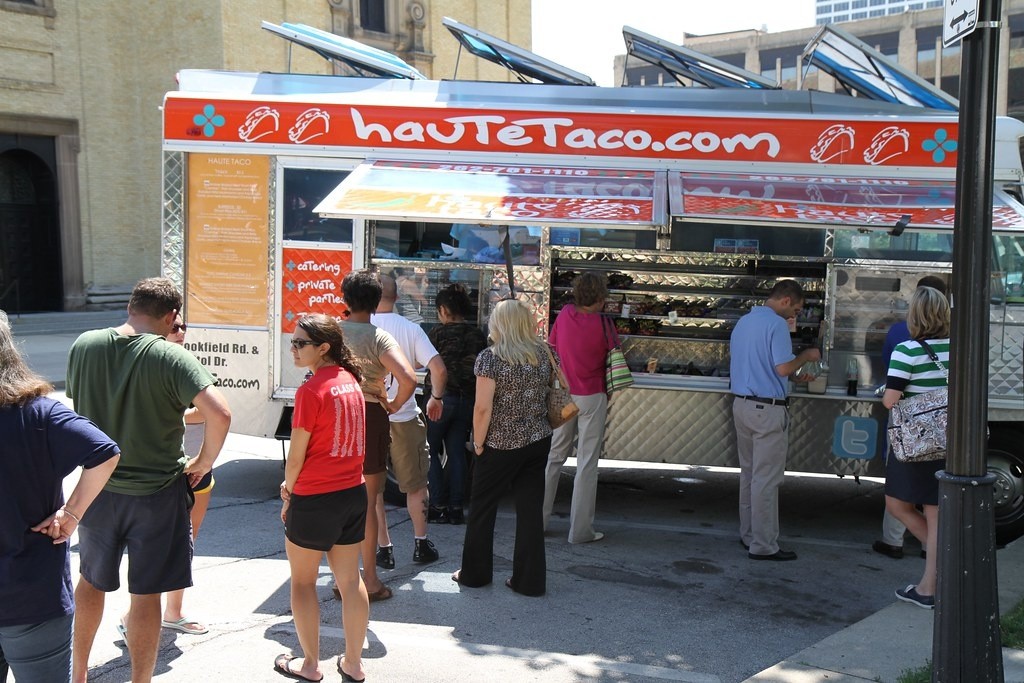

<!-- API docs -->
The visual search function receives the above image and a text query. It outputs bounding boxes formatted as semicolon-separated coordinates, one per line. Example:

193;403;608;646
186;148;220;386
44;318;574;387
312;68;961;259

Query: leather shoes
873;540;902;559
749;550;796;560
739;539;751;550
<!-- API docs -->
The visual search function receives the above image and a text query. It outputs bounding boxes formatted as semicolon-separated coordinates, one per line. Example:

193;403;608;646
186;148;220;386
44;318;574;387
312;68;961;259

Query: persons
66;277;233;682
390;267;431;326
880;287;949;610
117;312;215;649
0;310;121;682
274;313;370;683
420;280;488;524
541;270;623;545
331;271;418;602
451;297;559;599
371;275;447;568
872;275;946;560
728;279;821;562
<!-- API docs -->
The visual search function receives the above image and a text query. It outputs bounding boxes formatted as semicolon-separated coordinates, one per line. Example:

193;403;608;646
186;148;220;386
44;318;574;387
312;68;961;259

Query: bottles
848;359;858;396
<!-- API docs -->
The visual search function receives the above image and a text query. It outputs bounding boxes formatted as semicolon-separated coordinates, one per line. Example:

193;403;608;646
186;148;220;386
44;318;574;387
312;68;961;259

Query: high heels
331;586;342;600
367;579;391;602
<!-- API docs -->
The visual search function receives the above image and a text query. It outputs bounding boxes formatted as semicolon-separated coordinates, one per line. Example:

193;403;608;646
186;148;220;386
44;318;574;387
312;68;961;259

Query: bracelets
473;442;484;449
430;392;443;400
64;509;80;523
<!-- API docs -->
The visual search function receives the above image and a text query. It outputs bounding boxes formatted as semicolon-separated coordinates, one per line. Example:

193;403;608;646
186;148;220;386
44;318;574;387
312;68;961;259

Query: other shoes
453;569;461;580
895;585;935;609
427;506;448;523
448;507;465;525
568;532;604;543
505;577;512;586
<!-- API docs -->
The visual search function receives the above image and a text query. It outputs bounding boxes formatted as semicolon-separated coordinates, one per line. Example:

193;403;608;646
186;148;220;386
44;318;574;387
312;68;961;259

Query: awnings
311;156;1024;269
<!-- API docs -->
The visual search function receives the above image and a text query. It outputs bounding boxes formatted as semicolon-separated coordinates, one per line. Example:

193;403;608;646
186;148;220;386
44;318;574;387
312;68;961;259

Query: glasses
291;340;322;349
170;323;186;333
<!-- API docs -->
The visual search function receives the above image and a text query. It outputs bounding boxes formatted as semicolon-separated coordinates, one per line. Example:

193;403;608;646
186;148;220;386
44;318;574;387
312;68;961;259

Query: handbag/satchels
542;344;580;429
888;339;990;461
601;315;634;394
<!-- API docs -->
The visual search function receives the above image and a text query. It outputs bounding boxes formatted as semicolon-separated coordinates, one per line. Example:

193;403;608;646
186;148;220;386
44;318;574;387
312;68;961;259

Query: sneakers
376;544;395;569
413;538;437;562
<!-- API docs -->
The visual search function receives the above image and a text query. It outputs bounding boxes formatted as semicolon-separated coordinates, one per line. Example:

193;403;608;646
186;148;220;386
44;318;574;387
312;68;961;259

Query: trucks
154;16;1024;542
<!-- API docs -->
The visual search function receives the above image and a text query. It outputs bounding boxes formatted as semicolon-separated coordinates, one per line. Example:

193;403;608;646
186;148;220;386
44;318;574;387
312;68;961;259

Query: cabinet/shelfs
553;262;825;347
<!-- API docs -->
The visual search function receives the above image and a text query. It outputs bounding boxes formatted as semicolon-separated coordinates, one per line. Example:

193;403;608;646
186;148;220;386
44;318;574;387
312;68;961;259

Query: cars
1002;253;1023;303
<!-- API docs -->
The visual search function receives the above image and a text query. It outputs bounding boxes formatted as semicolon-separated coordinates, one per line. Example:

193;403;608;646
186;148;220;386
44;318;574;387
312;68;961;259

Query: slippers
116;624;127;646
275;654;323;682
338;653;365;683
161;616;208;634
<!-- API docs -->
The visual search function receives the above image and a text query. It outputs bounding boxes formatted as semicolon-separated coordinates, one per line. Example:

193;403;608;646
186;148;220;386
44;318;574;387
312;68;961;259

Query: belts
736;394;788;406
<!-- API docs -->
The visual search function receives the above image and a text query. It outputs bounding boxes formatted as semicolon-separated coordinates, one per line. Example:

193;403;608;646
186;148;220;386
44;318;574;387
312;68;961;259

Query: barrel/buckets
807;367;831;395
